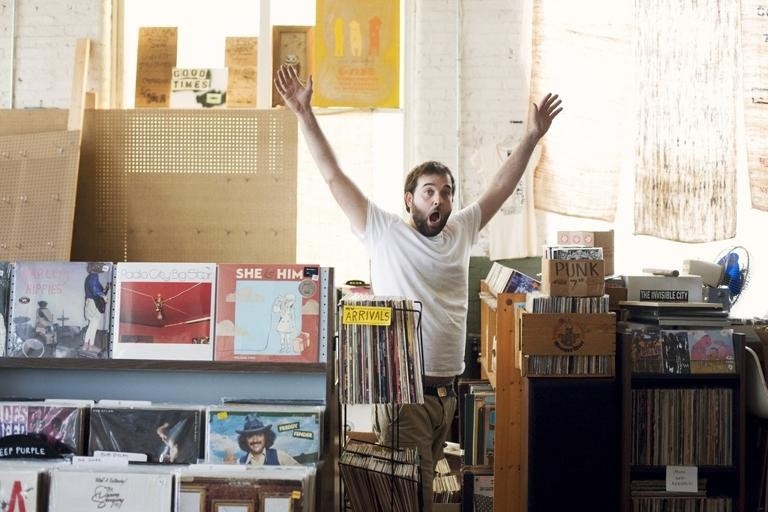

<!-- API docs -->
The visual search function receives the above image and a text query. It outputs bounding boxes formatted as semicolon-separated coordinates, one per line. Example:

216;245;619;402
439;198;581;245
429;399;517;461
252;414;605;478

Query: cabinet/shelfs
3;264;339;512
475;280;745;509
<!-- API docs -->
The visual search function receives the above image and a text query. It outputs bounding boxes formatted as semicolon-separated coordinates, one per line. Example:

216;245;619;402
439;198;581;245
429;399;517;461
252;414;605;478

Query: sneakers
81;343;103;354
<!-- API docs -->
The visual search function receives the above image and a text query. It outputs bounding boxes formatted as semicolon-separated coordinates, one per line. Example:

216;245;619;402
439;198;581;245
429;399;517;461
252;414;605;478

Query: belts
424;381;457;402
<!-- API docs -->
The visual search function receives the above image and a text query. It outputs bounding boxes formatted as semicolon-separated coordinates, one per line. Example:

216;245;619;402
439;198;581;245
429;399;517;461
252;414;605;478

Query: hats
235;412;273;436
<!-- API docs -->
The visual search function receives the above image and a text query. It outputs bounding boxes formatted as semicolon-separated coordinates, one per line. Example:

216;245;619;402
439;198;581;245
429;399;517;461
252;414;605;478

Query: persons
274;64;563;512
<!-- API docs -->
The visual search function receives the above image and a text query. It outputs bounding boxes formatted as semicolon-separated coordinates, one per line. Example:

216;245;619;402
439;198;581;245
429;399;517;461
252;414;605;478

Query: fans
680;242;752;310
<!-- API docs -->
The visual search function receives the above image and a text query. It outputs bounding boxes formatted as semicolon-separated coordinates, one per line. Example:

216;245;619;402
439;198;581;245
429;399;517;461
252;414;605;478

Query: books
1;398;326;511
484;243;734;378
454;379;496;511
334;284;424;511
630;387;736;511
1;261;321;364
